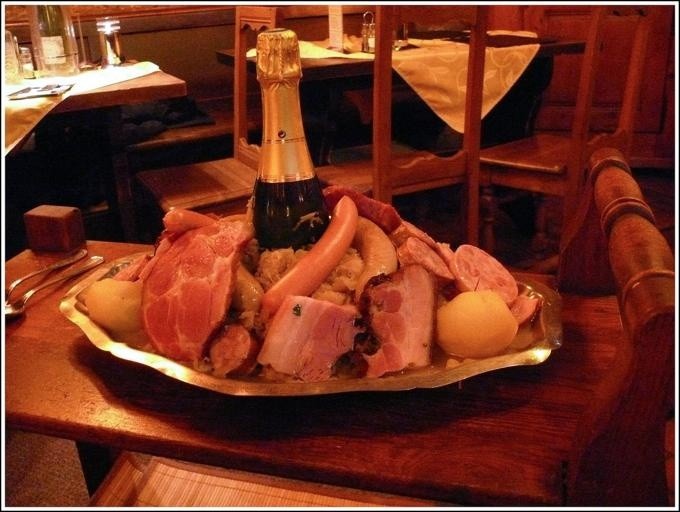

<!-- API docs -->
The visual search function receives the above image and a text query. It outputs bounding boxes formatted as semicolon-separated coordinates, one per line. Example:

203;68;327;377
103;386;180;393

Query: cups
94;15;125;67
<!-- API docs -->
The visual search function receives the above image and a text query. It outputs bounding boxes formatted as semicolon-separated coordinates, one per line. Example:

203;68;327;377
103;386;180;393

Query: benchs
5;6;256;155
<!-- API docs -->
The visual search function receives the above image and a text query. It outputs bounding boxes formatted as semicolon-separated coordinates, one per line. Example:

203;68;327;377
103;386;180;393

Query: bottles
250;26;330;251
360;21;375;54
6;5;82;86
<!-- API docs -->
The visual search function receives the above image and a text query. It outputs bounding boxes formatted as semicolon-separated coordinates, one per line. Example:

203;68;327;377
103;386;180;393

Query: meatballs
84;277;143;334
435;289;519;358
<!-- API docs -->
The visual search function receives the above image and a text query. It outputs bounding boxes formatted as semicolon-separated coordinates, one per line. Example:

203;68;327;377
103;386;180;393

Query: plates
57;248;564;397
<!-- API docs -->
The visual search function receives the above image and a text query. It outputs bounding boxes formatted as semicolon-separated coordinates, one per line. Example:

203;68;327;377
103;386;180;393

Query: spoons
6;246;103;324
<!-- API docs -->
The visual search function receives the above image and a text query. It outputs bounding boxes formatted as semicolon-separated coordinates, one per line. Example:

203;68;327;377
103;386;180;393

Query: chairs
482;4;672;292
294;6;488;250
131;6;286;222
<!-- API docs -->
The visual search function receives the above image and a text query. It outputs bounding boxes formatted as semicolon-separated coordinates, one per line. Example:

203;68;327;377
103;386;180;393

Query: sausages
231;262;264;314
262;194;358;318
162;208;216;235
352;216;398;312
